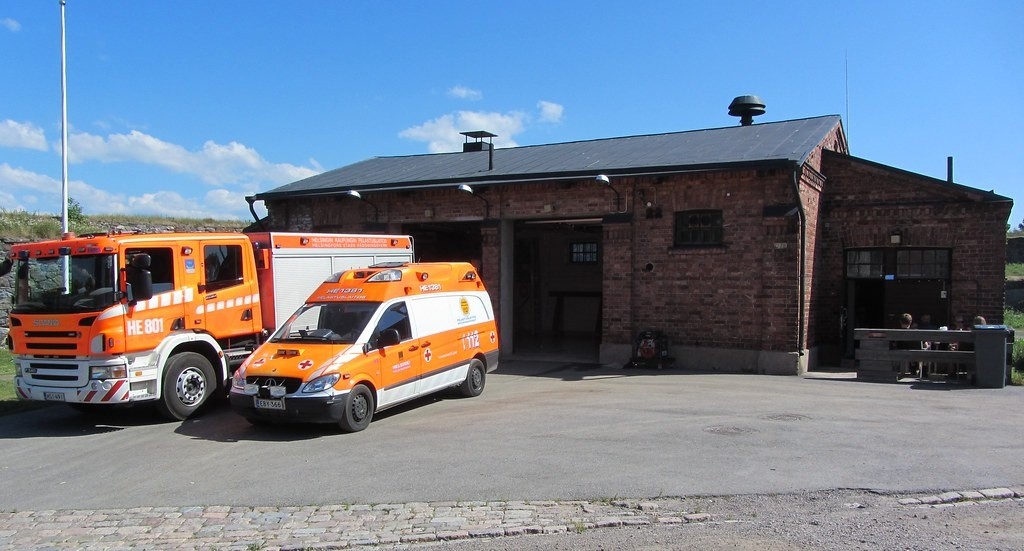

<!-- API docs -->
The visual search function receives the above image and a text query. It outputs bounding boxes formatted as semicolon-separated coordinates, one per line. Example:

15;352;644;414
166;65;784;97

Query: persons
955;315;986;376
895;310;939;374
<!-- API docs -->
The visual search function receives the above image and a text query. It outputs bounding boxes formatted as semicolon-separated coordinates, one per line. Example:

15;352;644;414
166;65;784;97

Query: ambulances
0;230;414;423
230;261;500;432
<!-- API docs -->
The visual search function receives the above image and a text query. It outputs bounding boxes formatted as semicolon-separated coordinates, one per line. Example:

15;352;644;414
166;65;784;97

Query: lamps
457;184;489;217
346;190;379;223
595;174;620;212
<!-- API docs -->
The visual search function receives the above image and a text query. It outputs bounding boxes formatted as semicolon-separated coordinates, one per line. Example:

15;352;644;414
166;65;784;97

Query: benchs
854;328;1014;384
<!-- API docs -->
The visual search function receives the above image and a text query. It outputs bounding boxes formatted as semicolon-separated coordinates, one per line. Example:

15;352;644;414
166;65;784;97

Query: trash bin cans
973;325;1009;389
